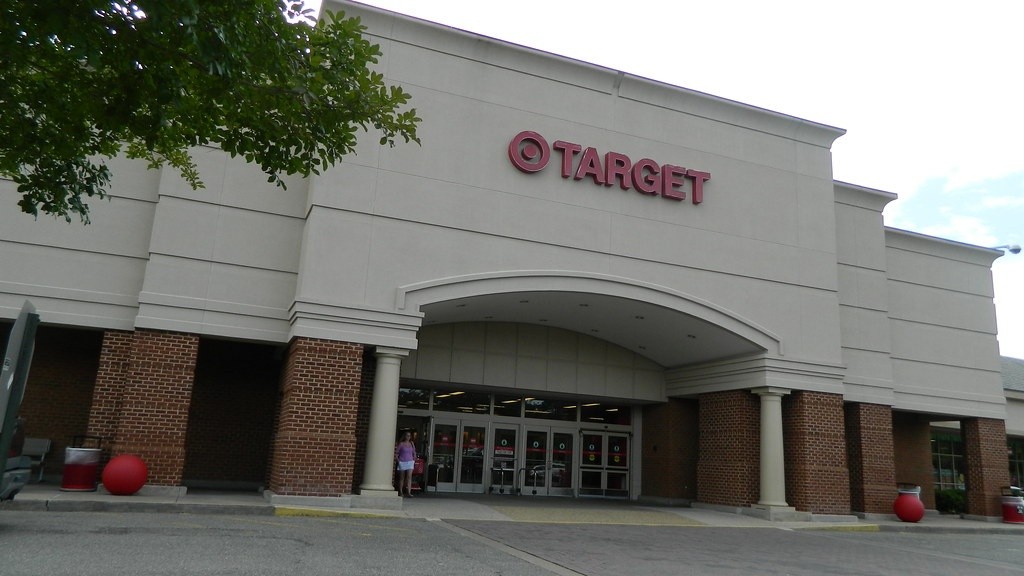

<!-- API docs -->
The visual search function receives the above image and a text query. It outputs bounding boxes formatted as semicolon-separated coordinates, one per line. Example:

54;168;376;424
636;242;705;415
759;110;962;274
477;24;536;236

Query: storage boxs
412;459;424;474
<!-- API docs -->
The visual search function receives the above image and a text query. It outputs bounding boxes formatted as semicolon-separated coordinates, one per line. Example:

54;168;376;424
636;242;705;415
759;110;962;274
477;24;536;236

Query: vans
551;463;567;479
529;464;546;479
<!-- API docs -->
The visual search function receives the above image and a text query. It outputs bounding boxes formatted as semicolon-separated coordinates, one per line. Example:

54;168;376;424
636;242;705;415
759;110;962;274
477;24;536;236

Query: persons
396;431;416;497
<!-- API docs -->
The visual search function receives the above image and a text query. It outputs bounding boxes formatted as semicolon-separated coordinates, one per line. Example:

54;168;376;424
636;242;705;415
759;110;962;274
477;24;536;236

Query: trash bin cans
1001;485;1024;523
896;482;921;500
60;445;103;492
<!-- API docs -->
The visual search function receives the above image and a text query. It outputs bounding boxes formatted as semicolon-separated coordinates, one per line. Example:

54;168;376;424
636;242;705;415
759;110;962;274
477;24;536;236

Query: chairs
19;438;51;483
